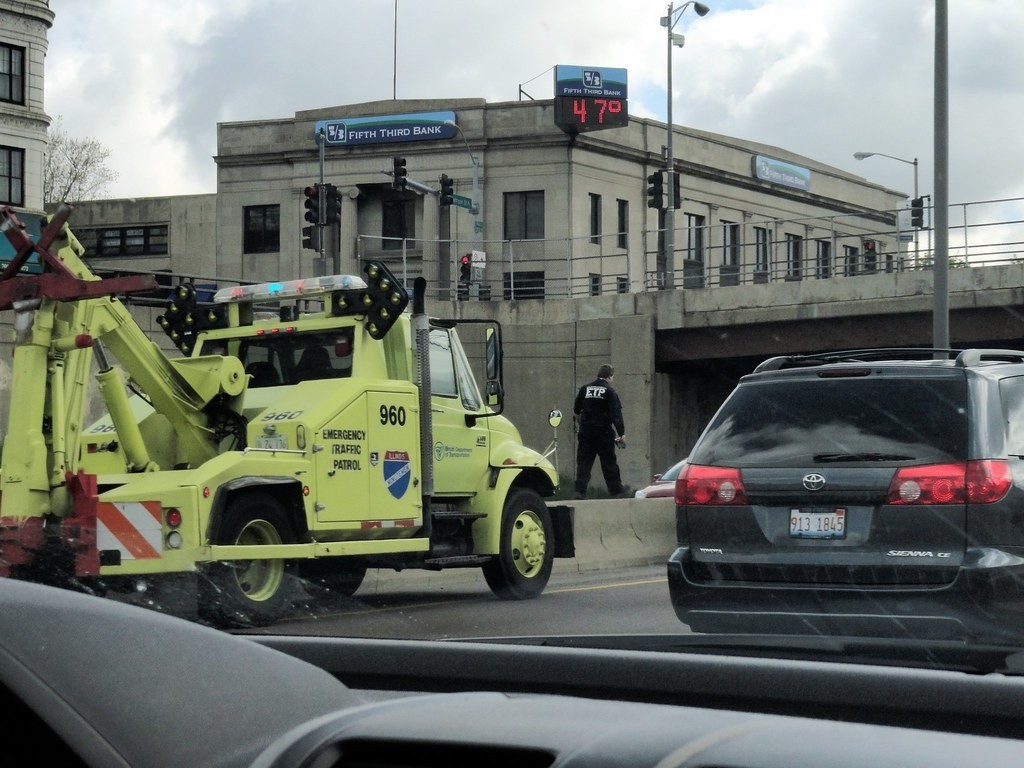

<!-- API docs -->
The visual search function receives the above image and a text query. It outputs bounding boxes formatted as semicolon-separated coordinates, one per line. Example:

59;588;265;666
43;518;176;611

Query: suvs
667;344;1024;676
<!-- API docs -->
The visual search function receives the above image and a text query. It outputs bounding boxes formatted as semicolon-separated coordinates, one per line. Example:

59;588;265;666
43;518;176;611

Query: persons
573;364;626;498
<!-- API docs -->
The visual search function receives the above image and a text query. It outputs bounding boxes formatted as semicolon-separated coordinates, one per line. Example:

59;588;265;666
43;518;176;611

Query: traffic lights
304;185;320;222
911;199;924;227
441;173;453;206
460;254;471;284
328;186;341;223
302;225;318;250
864;241;874;268
647;172;663;209
392;155;407;190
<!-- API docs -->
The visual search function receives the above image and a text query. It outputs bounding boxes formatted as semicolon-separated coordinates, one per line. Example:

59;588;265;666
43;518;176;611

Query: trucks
0;201;561;626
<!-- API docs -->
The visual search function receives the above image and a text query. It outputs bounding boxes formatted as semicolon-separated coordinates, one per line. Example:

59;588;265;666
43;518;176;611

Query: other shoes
613;491;626;499
573;492;589;501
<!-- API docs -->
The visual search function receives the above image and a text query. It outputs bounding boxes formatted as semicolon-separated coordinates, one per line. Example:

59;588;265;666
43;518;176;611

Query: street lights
853;151;919;270
663;2;709;288
444;119;481;298
